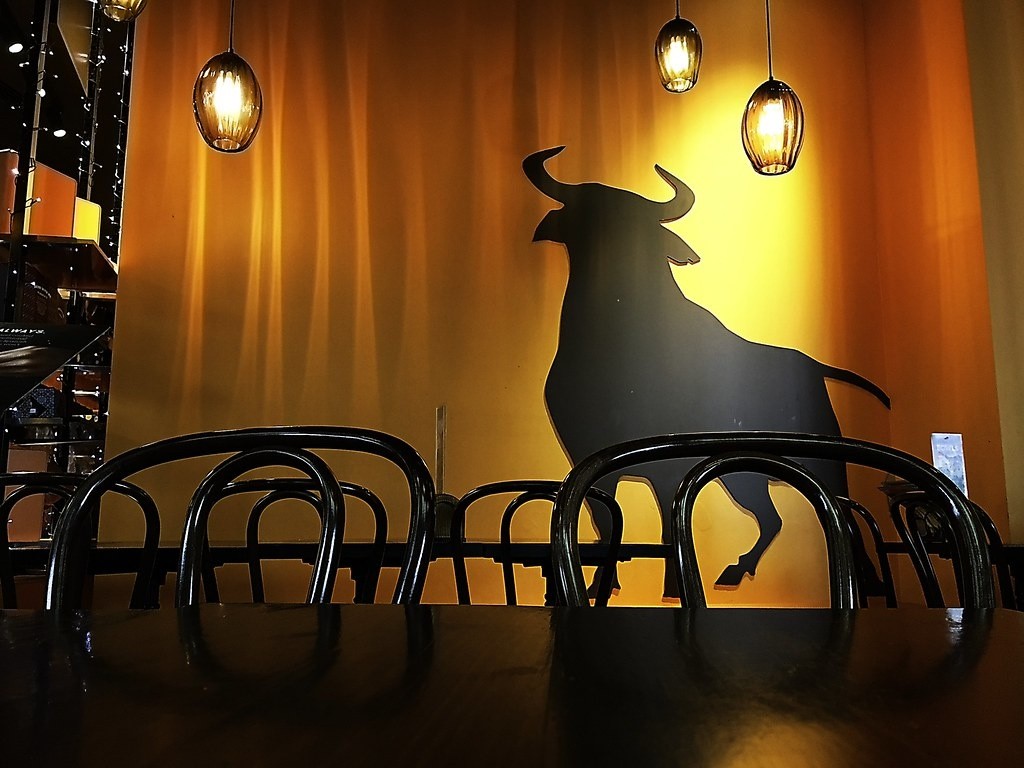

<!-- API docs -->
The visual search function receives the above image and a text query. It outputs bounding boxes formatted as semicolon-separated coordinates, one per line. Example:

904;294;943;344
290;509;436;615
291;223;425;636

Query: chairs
199;476;386;602
45;425;437;604
450;480;622;605
882;480;1013;603
835;493;898;604
0;478;161;607
547;431;995;606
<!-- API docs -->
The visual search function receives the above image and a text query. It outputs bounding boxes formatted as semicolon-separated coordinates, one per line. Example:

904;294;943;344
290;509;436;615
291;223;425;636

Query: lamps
741;0;806;177
191;0;264;153
655;0;704;94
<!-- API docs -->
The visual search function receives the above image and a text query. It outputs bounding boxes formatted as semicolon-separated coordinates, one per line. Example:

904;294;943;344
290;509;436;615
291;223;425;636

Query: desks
882;541;1024;613
0;605;1024;768
8;540;672;606
